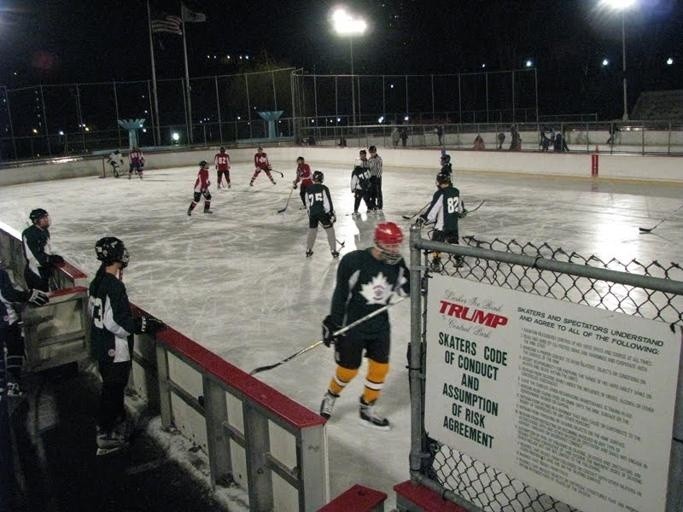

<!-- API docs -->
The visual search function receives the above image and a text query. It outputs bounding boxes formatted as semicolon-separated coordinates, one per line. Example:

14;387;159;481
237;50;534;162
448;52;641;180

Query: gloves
325;318;346;347
48;253;65;263
28;288;49;306
140;315;169;334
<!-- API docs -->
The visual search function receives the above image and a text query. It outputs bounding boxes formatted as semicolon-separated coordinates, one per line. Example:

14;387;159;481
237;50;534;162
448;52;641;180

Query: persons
293;156;339;258
316;223;410;432
250;146;278;187
1;266;49;396
392;127;409;147
214;147;233;190
437;125;443;145
417;153;464;273
338;135;347;146
510;125;569;153
295;134;315;146
107;147;146;180
607;123;620;144
22;209;64;292
88;236;166;448
351;145;384;221
187;161;213;217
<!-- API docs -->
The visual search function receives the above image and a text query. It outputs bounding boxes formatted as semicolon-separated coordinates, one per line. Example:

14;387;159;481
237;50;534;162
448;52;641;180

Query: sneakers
96;430;129;448
218;185;231;189
332;250;339;256
7;383;24;397
204;209;213;213
272;181;277;185
305;249;314;254
114;173;143;179
250;182;254;187
351;206;383;216
187;210;192;216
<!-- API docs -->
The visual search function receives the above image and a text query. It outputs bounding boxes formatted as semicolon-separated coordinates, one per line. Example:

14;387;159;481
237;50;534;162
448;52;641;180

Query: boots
319;391;339;421
357;396;390;428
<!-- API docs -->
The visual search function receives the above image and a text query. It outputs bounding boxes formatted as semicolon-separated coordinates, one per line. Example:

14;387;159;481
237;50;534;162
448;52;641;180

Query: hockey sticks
318;222;345;246
278;187;293;214
269;169;283;178
402;201;431;219
639;205;683;232
249;294;411;376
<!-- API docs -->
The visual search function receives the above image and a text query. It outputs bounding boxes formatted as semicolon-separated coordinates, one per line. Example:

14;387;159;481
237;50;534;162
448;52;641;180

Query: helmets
436;172;451;184
258;146;264;152
296;156;304;163
312;170;324;182
441;155;451;162
220;146;227;153
95;237;129;264
29;208;48;222
199;159;208;168
353;146;377;167
374;221;404;260
